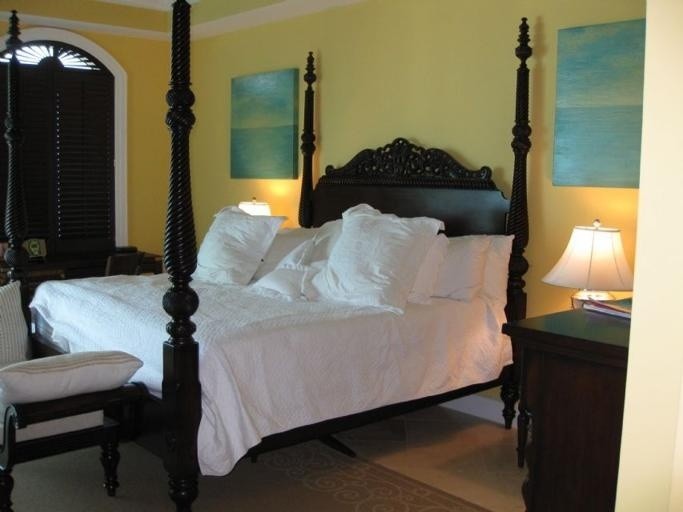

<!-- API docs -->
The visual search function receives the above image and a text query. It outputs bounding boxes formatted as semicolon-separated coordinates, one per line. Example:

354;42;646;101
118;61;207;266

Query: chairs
105;251;145;277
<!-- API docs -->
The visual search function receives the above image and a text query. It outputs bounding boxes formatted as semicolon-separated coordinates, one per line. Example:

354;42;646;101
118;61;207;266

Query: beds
2;0;532;512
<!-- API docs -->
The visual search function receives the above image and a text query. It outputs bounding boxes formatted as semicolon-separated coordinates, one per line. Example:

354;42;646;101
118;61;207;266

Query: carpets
0;438;496;511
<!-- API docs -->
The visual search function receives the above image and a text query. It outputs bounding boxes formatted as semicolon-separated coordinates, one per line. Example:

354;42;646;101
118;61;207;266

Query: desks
502;297;633;512
1;249;163;300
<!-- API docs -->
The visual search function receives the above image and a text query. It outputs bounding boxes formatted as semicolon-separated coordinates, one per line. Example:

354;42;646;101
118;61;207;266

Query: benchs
0;280;150;511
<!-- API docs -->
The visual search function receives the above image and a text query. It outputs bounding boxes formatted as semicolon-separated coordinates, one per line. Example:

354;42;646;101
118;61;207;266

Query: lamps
237;197;271;216
539;219;633;311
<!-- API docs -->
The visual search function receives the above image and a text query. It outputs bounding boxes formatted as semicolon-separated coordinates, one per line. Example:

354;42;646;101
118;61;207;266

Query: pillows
1;281;145;444
295;218;449;305
430;234;491;302
191;205;287;286
311;203;446;315
477;235;515;307
243;228;316;302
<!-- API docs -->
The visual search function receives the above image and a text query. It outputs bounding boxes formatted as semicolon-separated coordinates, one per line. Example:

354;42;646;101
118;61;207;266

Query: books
582;297;632;321
